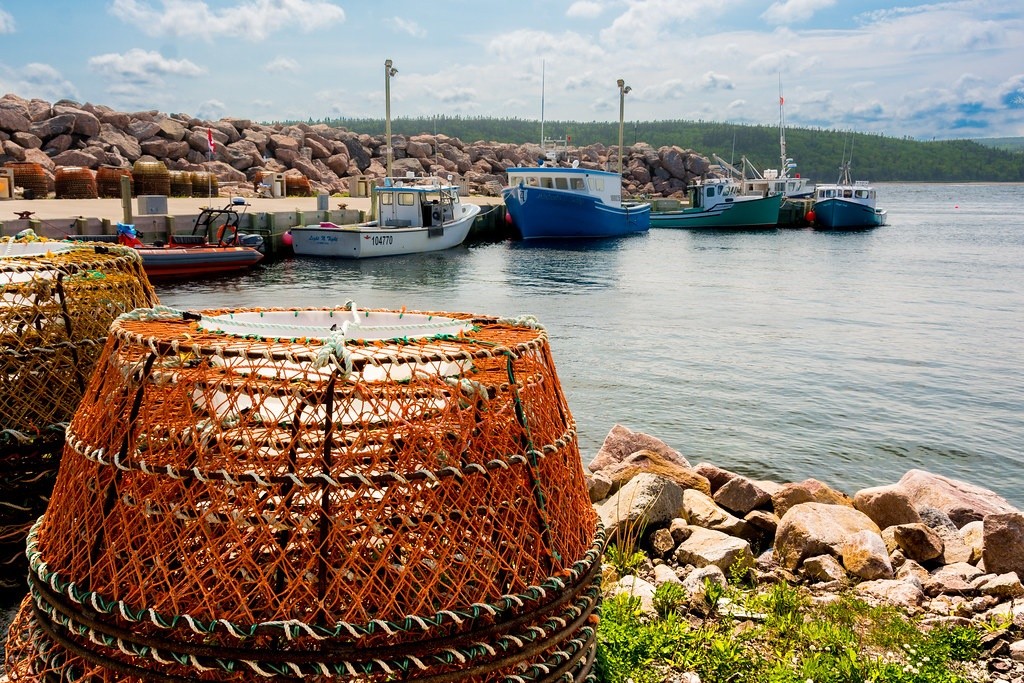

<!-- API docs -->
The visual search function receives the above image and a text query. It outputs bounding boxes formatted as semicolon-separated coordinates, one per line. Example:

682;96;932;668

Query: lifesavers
217;224;238;247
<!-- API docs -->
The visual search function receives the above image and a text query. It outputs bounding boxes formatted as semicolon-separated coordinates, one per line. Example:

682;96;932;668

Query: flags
207;128;216;154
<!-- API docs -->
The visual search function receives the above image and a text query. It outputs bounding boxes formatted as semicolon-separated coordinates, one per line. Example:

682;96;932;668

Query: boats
95;239;264;274
648;176;780;230
501;166;655;240
749;176;815;199
291;169;483;259
811;181;890;230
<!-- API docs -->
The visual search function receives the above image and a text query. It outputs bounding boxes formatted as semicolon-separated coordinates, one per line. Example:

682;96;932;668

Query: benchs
65;233;118;243
171;234;204;244
